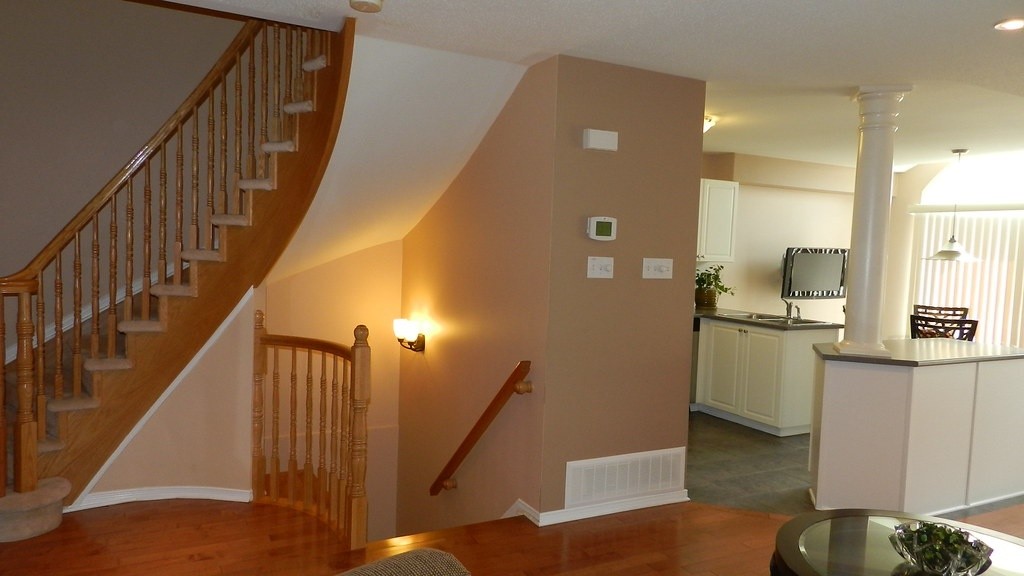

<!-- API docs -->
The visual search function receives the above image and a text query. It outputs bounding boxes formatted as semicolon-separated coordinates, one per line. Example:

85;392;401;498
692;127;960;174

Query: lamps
394;319;425;352
927;149;978;260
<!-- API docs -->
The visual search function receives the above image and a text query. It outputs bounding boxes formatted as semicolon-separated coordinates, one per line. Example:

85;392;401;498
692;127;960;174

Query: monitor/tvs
781;247;849;298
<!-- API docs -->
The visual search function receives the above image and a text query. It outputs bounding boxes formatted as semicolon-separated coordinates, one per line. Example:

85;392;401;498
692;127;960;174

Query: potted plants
695;264;735;309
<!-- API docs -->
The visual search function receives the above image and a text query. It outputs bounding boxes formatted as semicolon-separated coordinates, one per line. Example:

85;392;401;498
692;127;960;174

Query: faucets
782;298;793;318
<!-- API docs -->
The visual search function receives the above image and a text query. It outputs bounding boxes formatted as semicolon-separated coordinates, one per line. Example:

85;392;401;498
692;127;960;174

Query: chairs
910;304;978;342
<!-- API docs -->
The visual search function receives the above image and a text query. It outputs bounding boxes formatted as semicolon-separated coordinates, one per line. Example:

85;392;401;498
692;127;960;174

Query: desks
770;509;1024;576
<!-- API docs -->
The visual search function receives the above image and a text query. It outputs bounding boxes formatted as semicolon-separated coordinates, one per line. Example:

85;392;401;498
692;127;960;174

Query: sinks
759;318;827;330
719;313;782;324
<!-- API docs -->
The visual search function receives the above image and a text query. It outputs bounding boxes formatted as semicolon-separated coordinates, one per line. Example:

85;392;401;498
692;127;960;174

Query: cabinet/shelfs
807;338;1024;519
696;318;788;428
696;178;738;262
